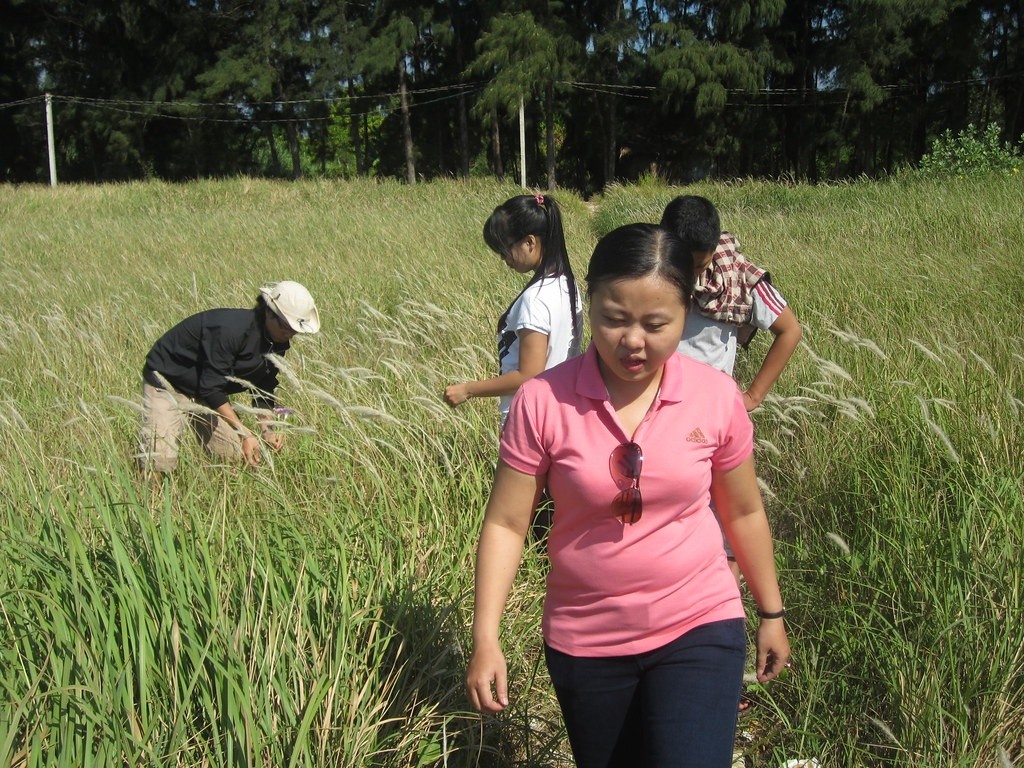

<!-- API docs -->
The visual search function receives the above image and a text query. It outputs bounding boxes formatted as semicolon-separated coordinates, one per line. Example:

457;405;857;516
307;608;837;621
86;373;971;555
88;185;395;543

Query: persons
442;193;585;560
662;198;803;414
144;281;322;488
465;224;793;768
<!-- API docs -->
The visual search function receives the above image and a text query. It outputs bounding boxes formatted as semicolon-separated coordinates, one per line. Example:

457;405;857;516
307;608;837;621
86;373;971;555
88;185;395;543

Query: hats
258;281;321;335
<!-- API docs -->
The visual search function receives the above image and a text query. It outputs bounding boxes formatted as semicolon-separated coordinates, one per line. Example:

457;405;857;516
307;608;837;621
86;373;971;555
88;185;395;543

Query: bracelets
756;605;788;621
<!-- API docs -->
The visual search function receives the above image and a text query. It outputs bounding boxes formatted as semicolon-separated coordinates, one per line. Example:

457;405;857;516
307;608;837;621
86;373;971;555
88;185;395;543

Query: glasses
275;314;299;337
612;442;643;526
